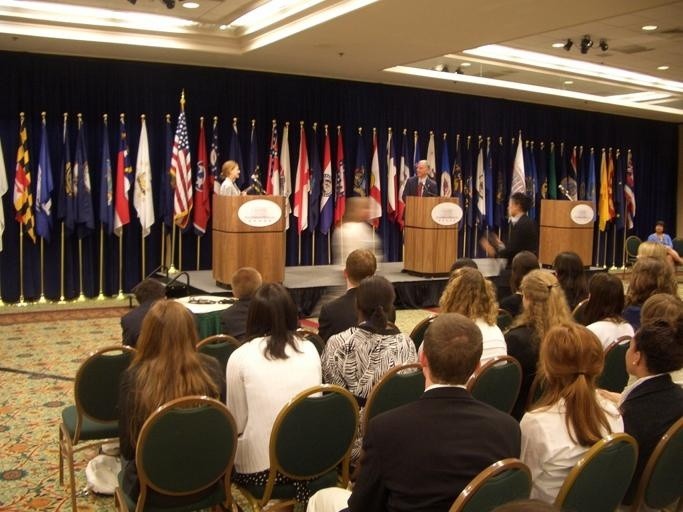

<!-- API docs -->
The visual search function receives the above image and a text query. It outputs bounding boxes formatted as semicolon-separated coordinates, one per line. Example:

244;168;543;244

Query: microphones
252;165;260;175
558;185;570;197
419;178;428;191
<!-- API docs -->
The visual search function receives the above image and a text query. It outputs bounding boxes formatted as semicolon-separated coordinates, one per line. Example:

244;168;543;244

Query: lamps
564;35;608;55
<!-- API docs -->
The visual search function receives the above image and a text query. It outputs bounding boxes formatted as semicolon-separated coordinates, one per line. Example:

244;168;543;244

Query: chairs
671;237;683;283
622;236;643;280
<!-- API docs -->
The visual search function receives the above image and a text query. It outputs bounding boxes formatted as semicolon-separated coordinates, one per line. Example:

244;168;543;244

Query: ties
417;182;423;195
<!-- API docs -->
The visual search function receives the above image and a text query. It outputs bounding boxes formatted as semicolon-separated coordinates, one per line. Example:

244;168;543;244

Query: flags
1;111;637;238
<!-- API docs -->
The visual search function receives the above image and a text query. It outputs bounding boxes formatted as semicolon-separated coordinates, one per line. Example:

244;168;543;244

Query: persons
647;221;683;277
480;191;537;303
118;242;682;512
330;196;382;283
